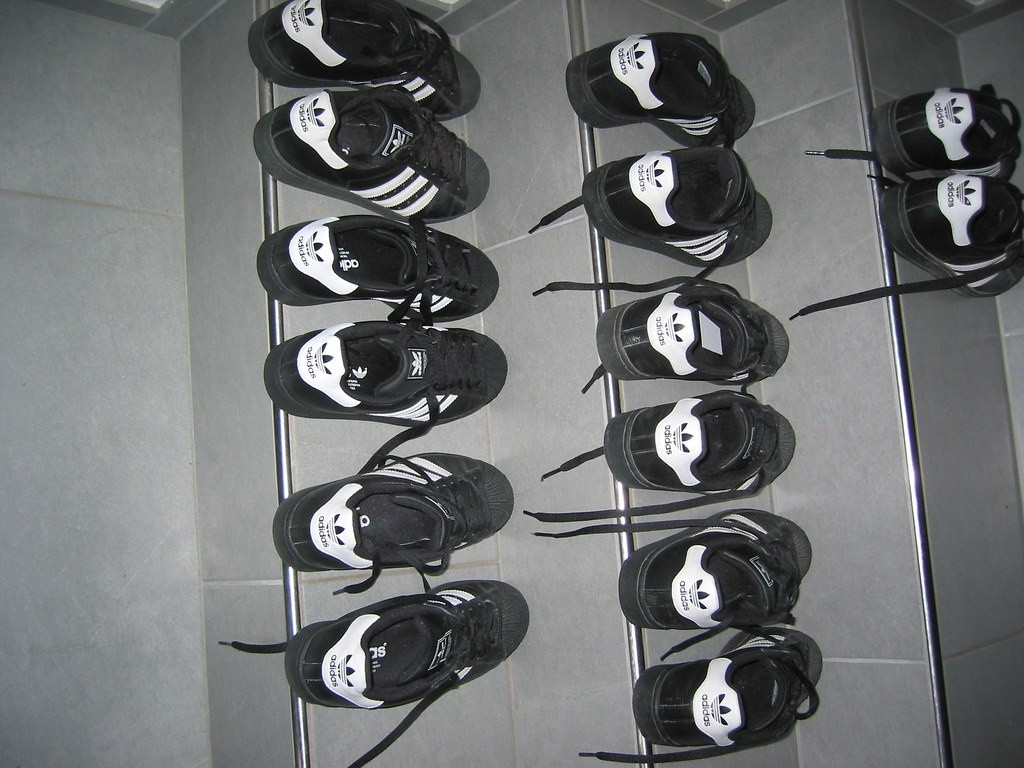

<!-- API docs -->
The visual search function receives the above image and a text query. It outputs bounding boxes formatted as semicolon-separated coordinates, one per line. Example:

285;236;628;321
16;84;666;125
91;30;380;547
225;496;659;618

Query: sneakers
805;84;1022;180
273;452;516;594
529;147;773;286
263;320;510;473
532;276;790;393
577;627;822;763
247;0;482;122
522;390;796;522
565;31;756;147
530;508;814;662
253;87;490;224
218;568;531;768
788;175;1024;319
257;215;501;325
